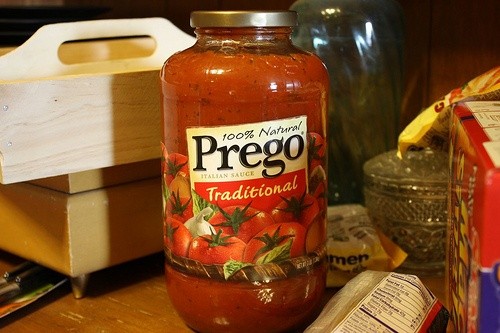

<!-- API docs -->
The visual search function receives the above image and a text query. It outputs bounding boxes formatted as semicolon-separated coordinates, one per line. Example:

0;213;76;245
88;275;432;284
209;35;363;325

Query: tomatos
159;133;327;264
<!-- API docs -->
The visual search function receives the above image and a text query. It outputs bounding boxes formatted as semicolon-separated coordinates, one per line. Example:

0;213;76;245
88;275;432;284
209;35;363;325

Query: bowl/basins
363;150;450;277
